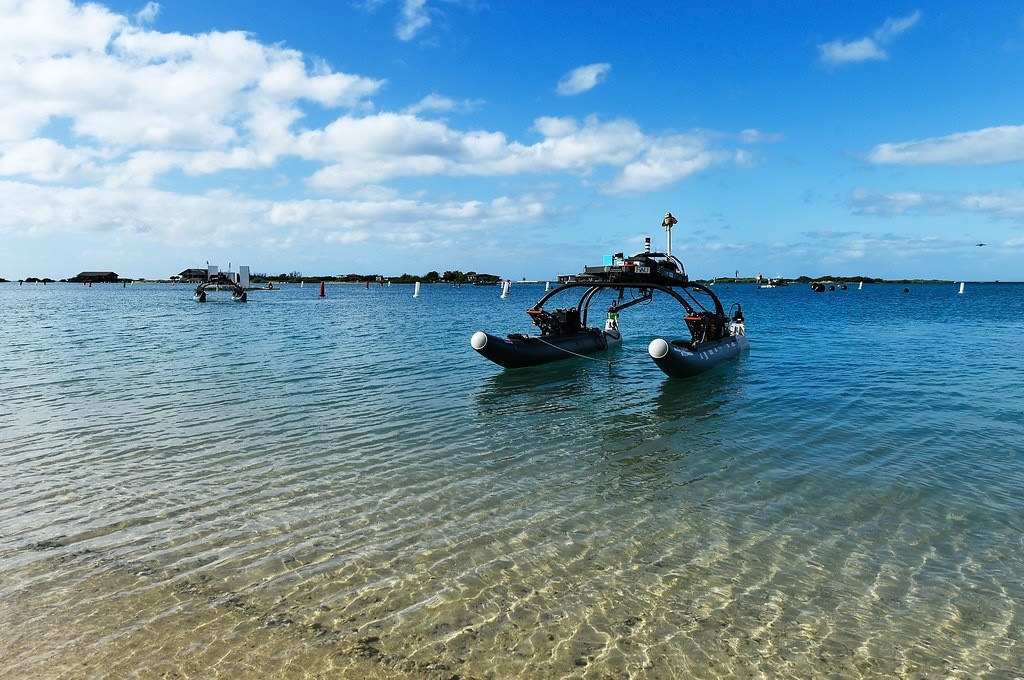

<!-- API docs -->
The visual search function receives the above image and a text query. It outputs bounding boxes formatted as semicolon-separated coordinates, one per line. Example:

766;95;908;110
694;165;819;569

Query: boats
192;273;247;304
469;213;750;376
